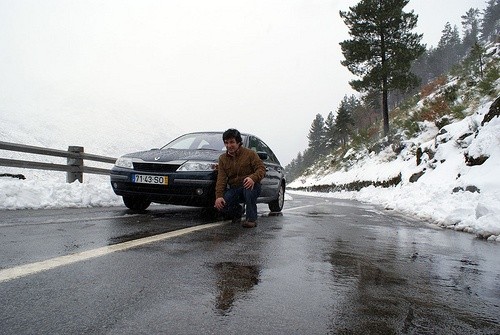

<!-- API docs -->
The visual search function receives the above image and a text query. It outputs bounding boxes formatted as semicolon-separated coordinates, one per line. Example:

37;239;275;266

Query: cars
110;131;287;218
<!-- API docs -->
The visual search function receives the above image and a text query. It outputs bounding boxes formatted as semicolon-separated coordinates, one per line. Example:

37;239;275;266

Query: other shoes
242;218;257;228
232;208;245;223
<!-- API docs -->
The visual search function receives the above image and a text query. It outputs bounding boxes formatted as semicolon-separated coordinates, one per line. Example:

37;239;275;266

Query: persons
214;129;266;228
